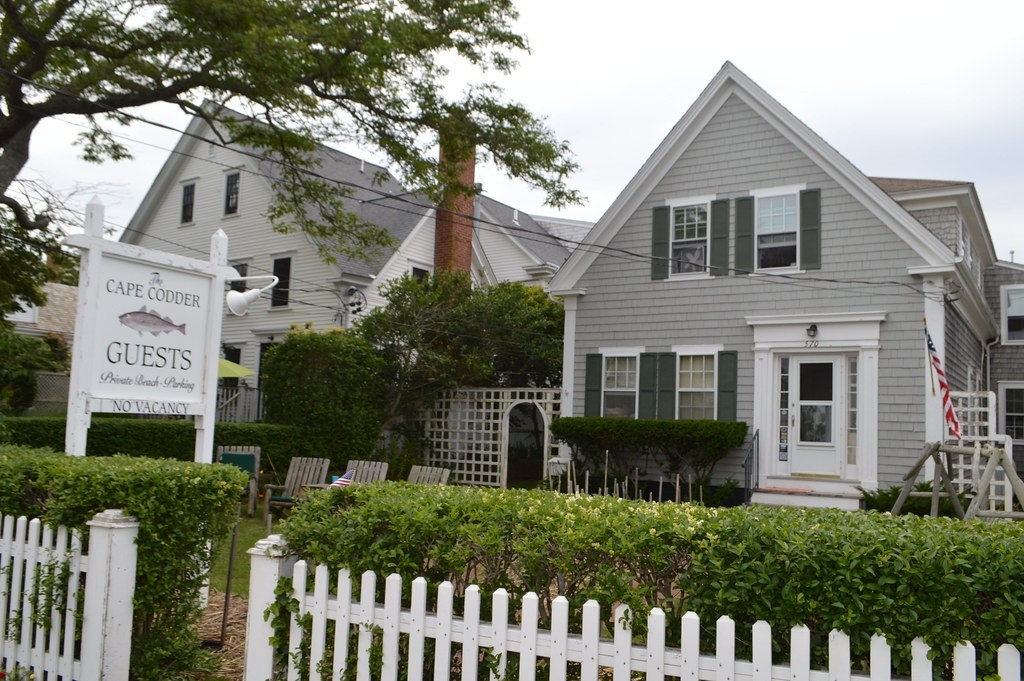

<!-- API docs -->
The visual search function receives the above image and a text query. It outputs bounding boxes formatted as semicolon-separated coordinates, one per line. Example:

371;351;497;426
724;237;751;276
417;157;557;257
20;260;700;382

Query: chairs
264;457;330;526
216;446;261;519
345;460;389;484
407;465;452;486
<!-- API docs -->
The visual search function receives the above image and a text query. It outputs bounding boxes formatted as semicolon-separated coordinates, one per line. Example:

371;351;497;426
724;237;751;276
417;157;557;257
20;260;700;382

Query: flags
327;469;353;489
927;325;962;441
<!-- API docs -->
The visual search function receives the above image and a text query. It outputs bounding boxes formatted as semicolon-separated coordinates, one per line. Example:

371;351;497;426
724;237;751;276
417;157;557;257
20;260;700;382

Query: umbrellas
218;358;258;378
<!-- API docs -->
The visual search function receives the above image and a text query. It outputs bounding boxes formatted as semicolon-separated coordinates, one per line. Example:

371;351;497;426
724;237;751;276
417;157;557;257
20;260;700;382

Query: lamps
807;325;817;337
224;274;279;317
